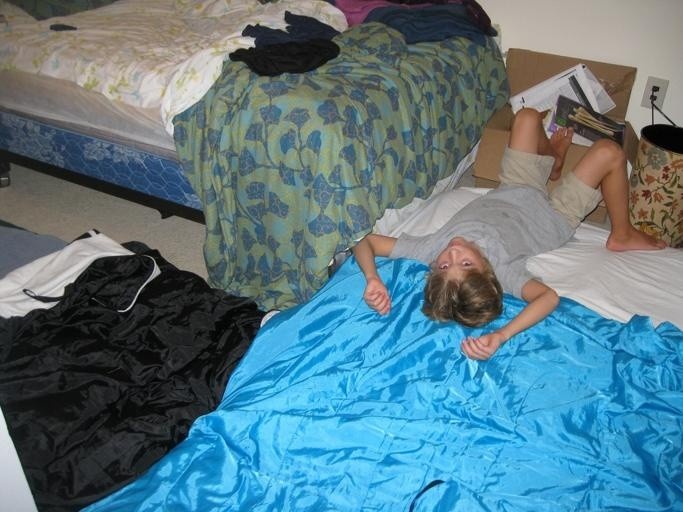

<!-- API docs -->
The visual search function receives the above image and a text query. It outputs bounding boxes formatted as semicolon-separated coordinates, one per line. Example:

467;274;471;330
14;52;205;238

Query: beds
1;219;70;283
0;0;507;227
78;184;683;512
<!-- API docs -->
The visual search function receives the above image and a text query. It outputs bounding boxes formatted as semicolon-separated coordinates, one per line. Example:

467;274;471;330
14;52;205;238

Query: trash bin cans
628;124;683;248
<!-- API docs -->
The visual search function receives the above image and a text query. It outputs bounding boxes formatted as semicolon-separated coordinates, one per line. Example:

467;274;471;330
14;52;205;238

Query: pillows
4;0;117;22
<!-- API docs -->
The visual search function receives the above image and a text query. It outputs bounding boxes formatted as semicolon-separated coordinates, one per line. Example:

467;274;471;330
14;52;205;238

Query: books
508;65;616;140
351;108;667;359
566;104;625;148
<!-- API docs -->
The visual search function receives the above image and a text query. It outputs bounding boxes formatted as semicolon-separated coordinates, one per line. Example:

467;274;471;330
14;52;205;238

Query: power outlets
638;75;670;113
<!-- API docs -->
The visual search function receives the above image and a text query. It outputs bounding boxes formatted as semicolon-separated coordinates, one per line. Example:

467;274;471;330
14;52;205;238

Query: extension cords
641;76;668;110
